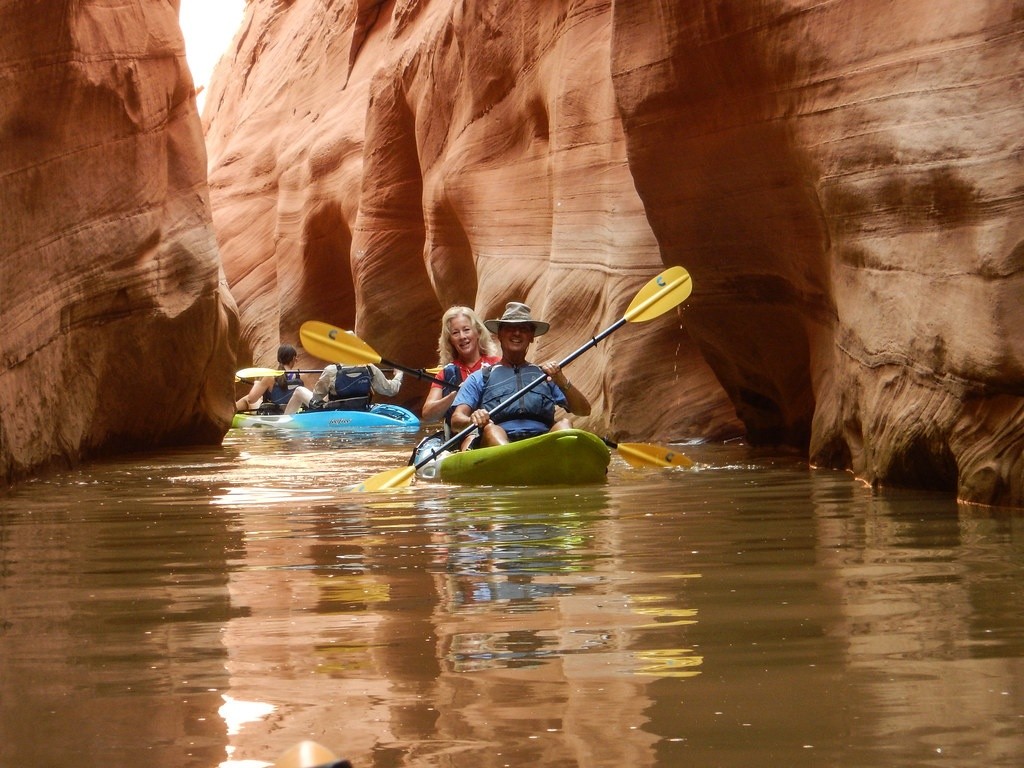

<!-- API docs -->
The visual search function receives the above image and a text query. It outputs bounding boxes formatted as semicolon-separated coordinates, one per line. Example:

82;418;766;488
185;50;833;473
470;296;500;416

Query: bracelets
559;378;571;392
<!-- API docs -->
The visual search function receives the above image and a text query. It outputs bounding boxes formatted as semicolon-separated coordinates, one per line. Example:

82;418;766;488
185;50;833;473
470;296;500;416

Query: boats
232;401;421;431
414;427;613;485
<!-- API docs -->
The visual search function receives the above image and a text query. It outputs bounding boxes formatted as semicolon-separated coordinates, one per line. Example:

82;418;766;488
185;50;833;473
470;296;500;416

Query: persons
284;331;403;416
235;345;306;415
450;302;591;452
422;306;503;451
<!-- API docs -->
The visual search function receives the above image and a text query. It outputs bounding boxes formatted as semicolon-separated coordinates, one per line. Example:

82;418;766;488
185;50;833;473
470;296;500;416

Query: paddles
235;364;447;379
352;263;695;495
234;374;254;385
299;321;696;473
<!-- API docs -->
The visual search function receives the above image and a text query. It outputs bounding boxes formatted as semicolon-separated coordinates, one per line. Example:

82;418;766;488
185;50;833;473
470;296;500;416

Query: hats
484;302;550;337
